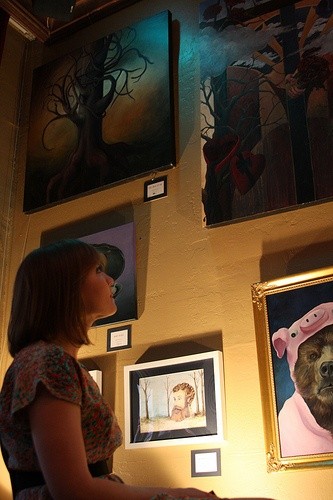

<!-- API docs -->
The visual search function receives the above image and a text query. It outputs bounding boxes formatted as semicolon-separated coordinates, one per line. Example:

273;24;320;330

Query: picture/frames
107;324;132;353
143;175;168;204
190;448;222;478
251;265;333;474
123;350;224;450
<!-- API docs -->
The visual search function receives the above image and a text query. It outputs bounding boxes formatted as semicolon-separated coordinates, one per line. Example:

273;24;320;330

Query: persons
0;237;222;500
